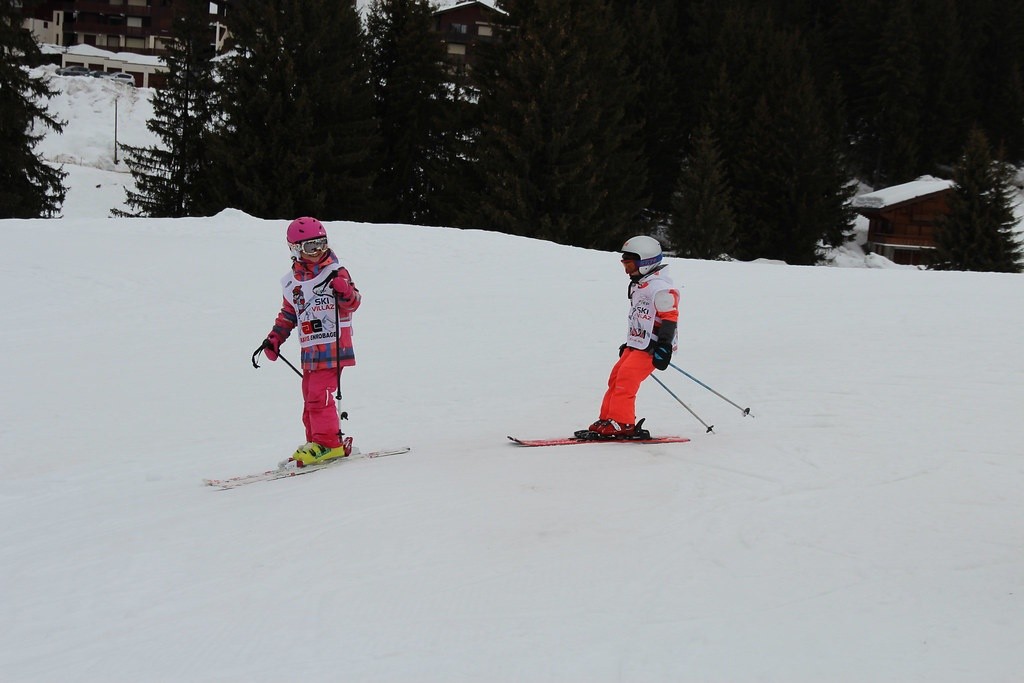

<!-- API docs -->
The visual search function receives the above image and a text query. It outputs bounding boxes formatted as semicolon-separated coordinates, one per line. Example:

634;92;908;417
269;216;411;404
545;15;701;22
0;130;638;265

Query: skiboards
507;434;692;447
201;436;411;490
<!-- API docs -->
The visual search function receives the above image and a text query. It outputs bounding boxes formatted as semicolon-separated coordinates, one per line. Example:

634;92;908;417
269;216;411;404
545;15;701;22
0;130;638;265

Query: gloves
264;337;281;361
332;276;352;295
653;342;672;370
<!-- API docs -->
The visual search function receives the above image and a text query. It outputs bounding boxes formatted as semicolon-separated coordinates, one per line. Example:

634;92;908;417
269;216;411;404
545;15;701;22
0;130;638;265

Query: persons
263;217;362;465
589;237;681;436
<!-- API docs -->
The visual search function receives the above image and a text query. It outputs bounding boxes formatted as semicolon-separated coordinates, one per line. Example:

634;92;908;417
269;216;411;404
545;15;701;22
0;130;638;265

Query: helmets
621;235;662;275
287;216;327;243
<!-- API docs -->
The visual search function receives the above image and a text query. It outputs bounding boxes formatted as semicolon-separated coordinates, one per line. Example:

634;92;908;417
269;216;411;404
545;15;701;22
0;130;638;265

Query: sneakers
589;419;636;436
293;442;344;465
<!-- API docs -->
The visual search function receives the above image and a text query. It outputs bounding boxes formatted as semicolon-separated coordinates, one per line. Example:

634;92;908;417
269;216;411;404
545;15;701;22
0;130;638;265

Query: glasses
621;260;639;274
300;238;328;255
